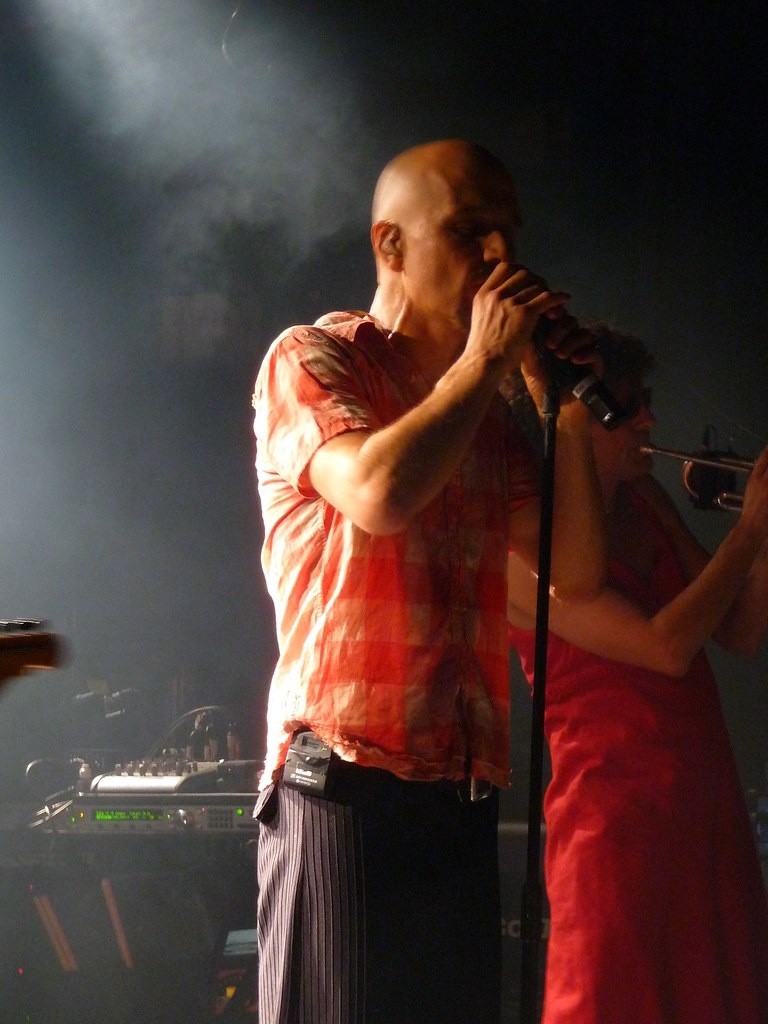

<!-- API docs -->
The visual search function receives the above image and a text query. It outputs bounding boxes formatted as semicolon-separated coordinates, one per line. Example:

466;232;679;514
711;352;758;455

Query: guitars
0;619;64;685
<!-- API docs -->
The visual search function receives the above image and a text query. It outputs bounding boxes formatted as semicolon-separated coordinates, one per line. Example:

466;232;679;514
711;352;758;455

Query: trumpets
640;440;756;512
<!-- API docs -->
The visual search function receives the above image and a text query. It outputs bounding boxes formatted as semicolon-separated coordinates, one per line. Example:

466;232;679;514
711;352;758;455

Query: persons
249;136;609;1024
503;326;768;1024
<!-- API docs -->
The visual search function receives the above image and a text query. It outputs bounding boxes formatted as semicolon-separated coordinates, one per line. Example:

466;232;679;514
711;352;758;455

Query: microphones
528;328;626;434
